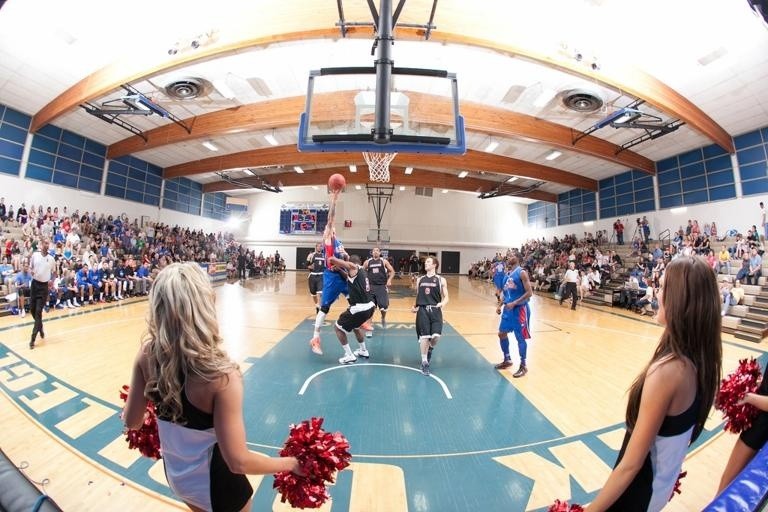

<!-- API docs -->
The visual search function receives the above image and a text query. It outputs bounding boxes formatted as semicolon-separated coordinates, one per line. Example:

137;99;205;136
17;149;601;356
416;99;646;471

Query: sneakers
354;349;369;358
361;321;373;331
495;362;513;368
365;331;373;337
81;302;85;306
310;337;323;355
381;317;386;329
106;297;110;302
513;365;527;377
422;362;430;376
109;296;114;301
89;300;93;304
339;356;357;364
427;347;433;362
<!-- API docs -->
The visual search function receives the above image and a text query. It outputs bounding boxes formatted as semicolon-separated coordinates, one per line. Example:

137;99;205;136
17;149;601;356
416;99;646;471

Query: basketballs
328;174;345;190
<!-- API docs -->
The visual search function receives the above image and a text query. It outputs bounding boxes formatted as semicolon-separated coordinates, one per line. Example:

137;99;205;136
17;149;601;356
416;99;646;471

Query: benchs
585;235;764;343
0;219;88;321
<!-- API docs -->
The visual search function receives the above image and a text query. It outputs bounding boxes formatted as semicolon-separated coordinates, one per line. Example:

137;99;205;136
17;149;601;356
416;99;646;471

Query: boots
640;307;646;315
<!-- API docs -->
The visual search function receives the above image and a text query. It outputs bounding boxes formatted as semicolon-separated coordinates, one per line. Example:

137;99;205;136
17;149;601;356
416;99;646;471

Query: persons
309;188;374;356
302;242;325;316
495;250;532;377
120;260;352;512
410;255;449;375
398;250;426;289
30;240;54;349
328;254;376;364
546;255;722;512
386;255;394;278
1;197;288;319
364;248;395;337
715;357;768;501
467;203;768;319
